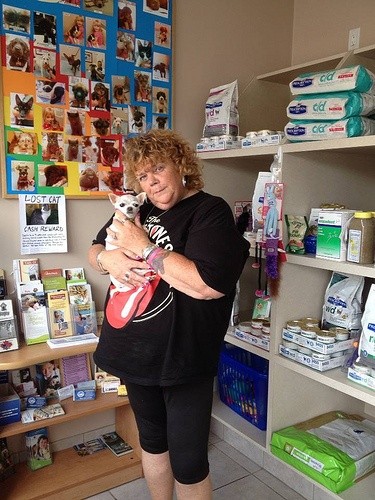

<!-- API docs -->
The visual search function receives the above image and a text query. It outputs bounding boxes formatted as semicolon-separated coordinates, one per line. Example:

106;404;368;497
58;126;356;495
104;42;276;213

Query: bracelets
142;244;159;259
96;249;107;272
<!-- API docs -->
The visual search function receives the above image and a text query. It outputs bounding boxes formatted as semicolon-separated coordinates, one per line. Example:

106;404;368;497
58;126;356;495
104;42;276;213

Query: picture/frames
0;0;176;200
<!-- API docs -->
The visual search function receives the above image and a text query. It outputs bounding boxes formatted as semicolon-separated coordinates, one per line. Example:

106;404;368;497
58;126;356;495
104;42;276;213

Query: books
25;426;52;470
0;258;98;347
0;438;17;481
73;438;103;456
13;355;121;424
100;431;133;456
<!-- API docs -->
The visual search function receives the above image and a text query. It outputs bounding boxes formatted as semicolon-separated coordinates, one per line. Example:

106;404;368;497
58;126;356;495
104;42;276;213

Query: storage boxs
196;134;290;152
218;327;270;431
316;211;349;263
279;327;360;371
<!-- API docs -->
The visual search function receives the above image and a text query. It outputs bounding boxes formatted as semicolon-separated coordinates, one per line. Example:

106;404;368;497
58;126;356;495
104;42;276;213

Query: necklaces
144;201;169;228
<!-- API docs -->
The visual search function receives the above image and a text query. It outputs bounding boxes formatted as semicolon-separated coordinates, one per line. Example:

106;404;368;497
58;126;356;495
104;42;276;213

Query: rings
122;218;129;223
126;270;131;277
114;233;117;239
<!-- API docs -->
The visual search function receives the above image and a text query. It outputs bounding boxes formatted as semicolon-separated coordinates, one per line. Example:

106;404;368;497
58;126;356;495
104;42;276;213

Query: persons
76;313;90;335
64;16;84;44
87;20;105;48
36;360;60;396
95;61;102;76
54;310;66;331
42;107;63;131
87;130;251;500
32;436;50;461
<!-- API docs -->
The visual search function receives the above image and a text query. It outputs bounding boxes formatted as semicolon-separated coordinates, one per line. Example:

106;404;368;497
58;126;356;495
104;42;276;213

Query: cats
43;132;64;162
2;10;30;33
30;204;52;225
67;110;83;136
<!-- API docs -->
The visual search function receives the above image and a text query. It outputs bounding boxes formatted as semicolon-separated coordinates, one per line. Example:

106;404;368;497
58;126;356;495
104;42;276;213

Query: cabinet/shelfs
193;44;375;500
0;333;144;500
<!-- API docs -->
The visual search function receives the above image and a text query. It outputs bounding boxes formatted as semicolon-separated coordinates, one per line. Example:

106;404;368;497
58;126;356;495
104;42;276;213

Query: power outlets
348;27;361;51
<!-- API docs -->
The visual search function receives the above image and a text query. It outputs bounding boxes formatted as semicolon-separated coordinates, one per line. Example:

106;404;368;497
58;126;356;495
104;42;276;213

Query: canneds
239;318;270;341
283;317;349;362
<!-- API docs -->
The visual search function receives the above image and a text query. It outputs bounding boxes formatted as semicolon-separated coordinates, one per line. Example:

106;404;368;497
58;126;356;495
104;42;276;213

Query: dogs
38;81;110;110
83;136;102;162
7;38;30;72
113;72;168;134
67;137;79;162
116;26;168;79
106;191;153;298
8;132;36;155
44;164;67;187
68;25;99;48
14;93;33;126
15;165;31;190
34;54;54;79
101;144;119;166
104;172;123;192
